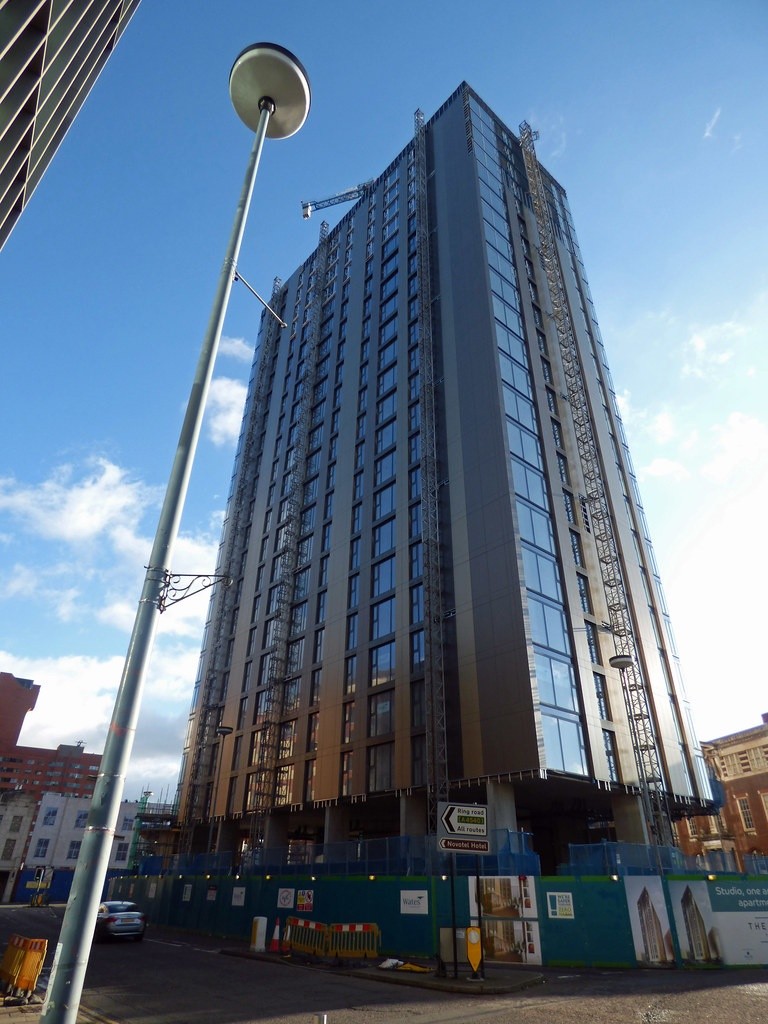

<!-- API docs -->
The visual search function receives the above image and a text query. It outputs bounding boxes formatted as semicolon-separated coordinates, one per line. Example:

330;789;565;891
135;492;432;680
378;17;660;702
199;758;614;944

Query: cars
94;901;148;942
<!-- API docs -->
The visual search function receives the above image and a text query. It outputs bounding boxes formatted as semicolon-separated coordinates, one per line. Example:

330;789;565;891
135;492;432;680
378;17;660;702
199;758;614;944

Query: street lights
606;654;664;878
31;40;317;1024
